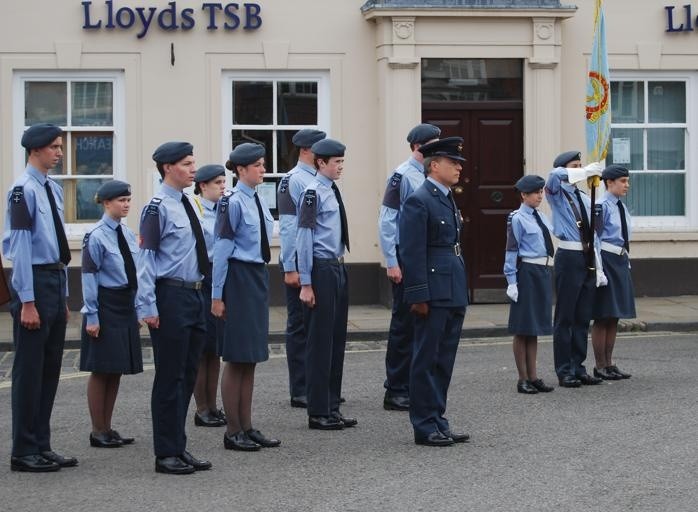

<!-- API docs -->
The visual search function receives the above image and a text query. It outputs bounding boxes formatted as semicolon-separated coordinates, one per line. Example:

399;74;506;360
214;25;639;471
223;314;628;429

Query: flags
587;0;611;190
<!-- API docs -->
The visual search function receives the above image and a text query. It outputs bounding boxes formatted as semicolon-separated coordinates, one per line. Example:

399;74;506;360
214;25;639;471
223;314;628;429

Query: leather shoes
308;415;345;430
517;380;539;394
246;429;281;447
594;366;622;379
89;432;123;448
575;373;602;384
155;455;195;474
41;451;78;467
530;379;554;393
179;450;212;471
10;454;60;472
558;374;583;387
383;395;410;411
415;431;454;446
610;365;631;378
331;410;358;426
111;429;134;445
224;431;262;451
212;408;227;424
290;395;308;407
194;409;225;426
441;429;469;443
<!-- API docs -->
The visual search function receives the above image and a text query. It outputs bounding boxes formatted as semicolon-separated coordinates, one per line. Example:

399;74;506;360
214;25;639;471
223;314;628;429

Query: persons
545;151;606;388
400;137;470;446
378;124;441;412
212;142;281;451
592;164;637;381
80;180;143;449
193;164;228;427
503;175;556;394
2;123;79;472
297;139;358;430
278;130;346;408
136;142;213;476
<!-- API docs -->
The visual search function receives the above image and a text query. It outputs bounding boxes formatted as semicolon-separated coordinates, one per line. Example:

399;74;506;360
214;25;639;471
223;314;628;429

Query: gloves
507;283;518;303
593;247;608;288
565;161;603;185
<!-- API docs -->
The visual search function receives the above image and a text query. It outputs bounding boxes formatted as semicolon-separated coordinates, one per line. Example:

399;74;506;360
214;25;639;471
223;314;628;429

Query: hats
417;137;466;162
602;165;628;179
230;144;265;166
554;151;581;168
193;165;225;183
21;123;63;148
407;124;441;144
95;181;132;202
311;139;346;157
292;128;326;146
153;142;193;163
515;175;545;192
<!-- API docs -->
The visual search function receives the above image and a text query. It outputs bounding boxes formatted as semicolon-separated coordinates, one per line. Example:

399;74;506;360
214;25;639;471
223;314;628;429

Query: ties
253;192;272;264
44;180;72;267
533;209;554;258
180;195;210;277
574;185;591;243
616;201;629;254
115;225;138;290
331;182;350;253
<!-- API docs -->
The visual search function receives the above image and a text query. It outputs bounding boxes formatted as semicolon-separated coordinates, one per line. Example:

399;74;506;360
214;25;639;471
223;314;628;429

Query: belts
330;257;344;266
437;244;462;256
159;277;202;290
558;240;584;251
521;256;554;267
33;262;64;271
599;240;627;256
